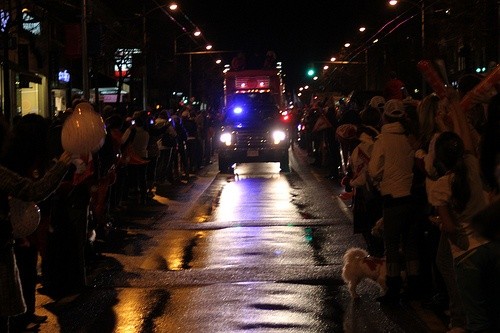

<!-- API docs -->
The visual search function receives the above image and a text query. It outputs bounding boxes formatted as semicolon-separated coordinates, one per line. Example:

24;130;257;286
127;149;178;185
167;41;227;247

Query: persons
288;60;500;333
0;102;227;333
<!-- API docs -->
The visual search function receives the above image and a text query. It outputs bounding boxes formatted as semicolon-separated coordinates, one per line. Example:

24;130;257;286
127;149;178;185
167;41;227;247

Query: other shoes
28;314;47;322
339;191;353;198
81;154;215;243
11;323;39;332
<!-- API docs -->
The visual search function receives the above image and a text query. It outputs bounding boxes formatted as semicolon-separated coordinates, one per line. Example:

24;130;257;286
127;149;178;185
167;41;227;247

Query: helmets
10;203;40;240
60;103;106;154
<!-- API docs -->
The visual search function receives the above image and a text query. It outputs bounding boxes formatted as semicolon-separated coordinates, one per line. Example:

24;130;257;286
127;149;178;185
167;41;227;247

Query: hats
383;98;403;117
370;97;384;108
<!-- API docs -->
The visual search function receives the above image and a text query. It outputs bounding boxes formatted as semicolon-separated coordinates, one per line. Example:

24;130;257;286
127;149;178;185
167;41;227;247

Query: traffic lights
306;67;315;77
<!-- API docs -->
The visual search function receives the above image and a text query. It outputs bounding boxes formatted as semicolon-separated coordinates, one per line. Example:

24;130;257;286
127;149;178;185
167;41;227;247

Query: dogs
341;247;387;300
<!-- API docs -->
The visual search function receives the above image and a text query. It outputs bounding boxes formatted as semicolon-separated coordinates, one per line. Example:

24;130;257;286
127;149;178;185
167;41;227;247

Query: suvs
217;91;296;171
